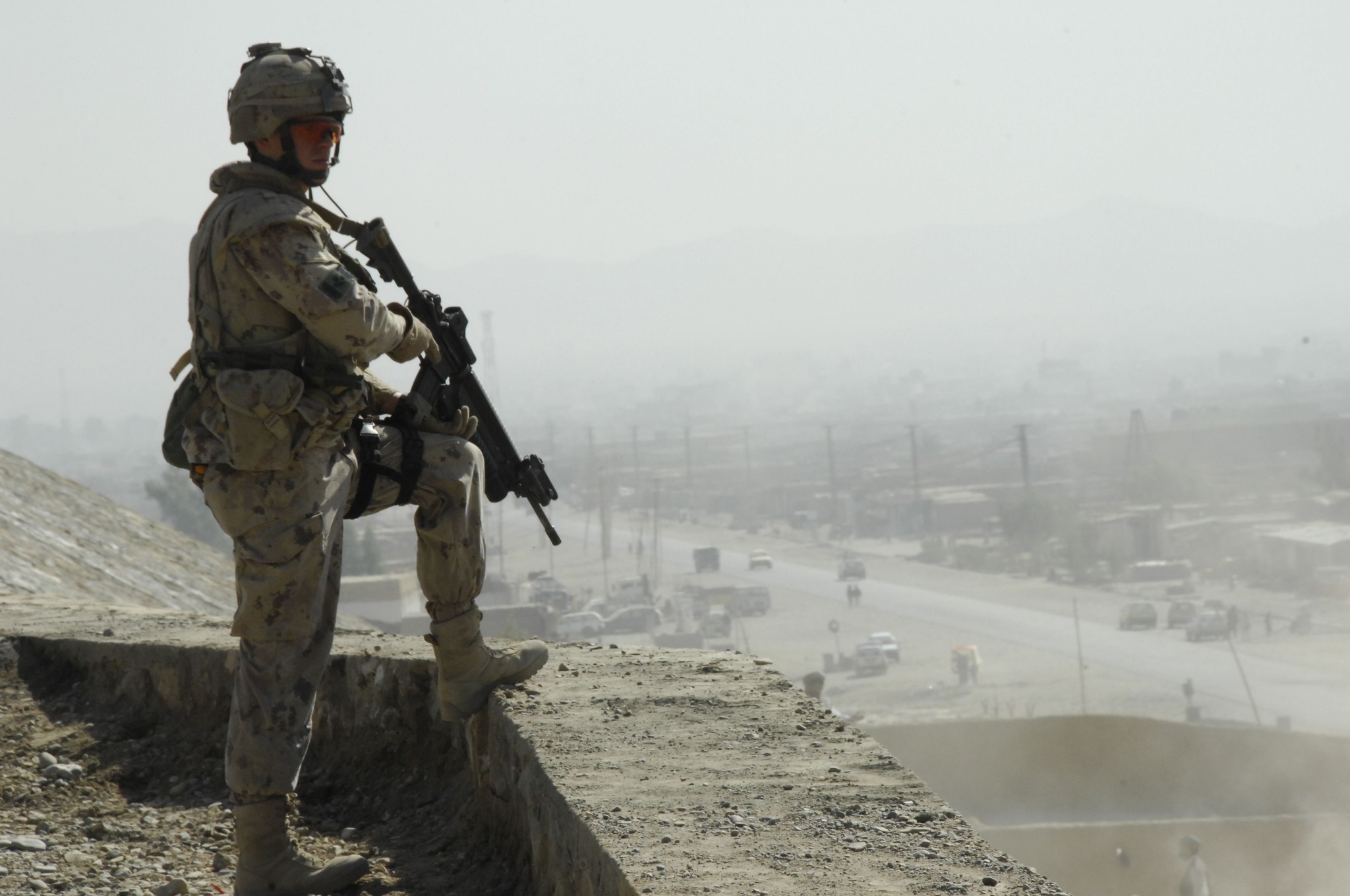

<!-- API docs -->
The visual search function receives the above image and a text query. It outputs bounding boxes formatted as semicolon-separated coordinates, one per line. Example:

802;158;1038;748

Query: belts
200;352;340;389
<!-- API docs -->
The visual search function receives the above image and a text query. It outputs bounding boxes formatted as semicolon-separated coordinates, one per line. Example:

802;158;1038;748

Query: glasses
288;114;342;143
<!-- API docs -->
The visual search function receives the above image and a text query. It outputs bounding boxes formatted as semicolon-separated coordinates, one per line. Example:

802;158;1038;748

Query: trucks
691;545;720;571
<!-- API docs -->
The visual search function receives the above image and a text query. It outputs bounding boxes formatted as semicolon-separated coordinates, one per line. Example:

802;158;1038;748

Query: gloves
385;302;442;364
395;395;479;441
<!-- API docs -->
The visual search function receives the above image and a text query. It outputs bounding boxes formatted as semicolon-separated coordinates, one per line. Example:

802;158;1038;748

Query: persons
847;584;862;604
163;43;548;896
1179;837;1209;896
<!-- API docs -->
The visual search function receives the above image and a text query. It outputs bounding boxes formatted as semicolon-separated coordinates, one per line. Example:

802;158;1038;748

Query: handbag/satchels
215;368;303;471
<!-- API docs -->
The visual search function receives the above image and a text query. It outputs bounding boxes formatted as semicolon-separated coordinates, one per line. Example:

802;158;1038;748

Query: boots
424;604;550;722
232;791;371;896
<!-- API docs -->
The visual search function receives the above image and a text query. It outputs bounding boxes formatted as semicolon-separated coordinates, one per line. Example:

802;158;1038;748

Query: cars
747;549;775;568
523;570;769;649
837;559;868;579
1119;601;1233;641
856;629;902;677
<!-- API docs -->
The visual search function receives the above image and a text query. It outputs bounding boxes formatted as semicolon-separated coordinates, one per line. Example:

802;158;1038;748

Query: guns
356;216;561;548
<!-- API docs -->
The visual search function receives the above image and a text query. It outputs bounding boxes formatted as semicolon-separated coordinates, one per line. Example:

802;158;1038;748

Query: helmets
227;49;351;144
